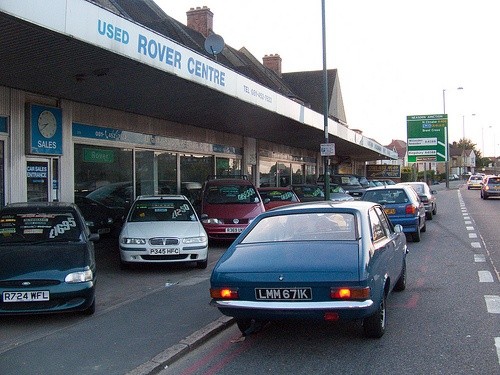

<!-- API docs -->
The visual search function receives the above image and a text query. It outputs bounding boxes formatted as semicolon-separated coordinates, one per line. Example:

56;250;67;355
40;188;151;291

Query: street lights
482;125;492;169
463;112;477;184
442;87;466;190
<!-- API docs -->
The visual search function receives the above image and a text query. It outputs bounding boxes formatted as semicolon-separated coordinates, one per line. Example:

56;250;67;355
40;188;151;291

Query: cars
359;183;429;242
479;175;500;200
466;175;484;190
316;181;355;201
181;181;203;201
117;193;210;271
26;193;126;240
256;185;300;211
207;199;410;339
462;171;472;176
449;173;459;181
0;198;100;318
82;179;198;217
476;172;486;177
287;184;328;202
395;181;437;220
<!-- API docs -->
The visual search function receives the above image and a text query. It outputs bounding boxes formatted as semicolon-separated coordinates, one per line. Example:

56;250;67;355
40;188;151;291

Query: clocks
39;112;57;138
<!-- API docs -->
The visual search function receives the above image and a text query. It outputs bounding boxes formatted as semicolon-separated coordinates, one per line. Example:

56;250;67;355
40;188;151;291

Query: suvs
316;173;396;196
197;174;267;243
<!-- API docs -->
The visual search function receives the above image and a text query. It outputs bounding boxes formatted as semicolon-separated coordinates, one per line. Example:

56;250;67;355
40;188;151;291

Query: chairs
396;193;406;203
208;190;223;202
49;216;78;241
